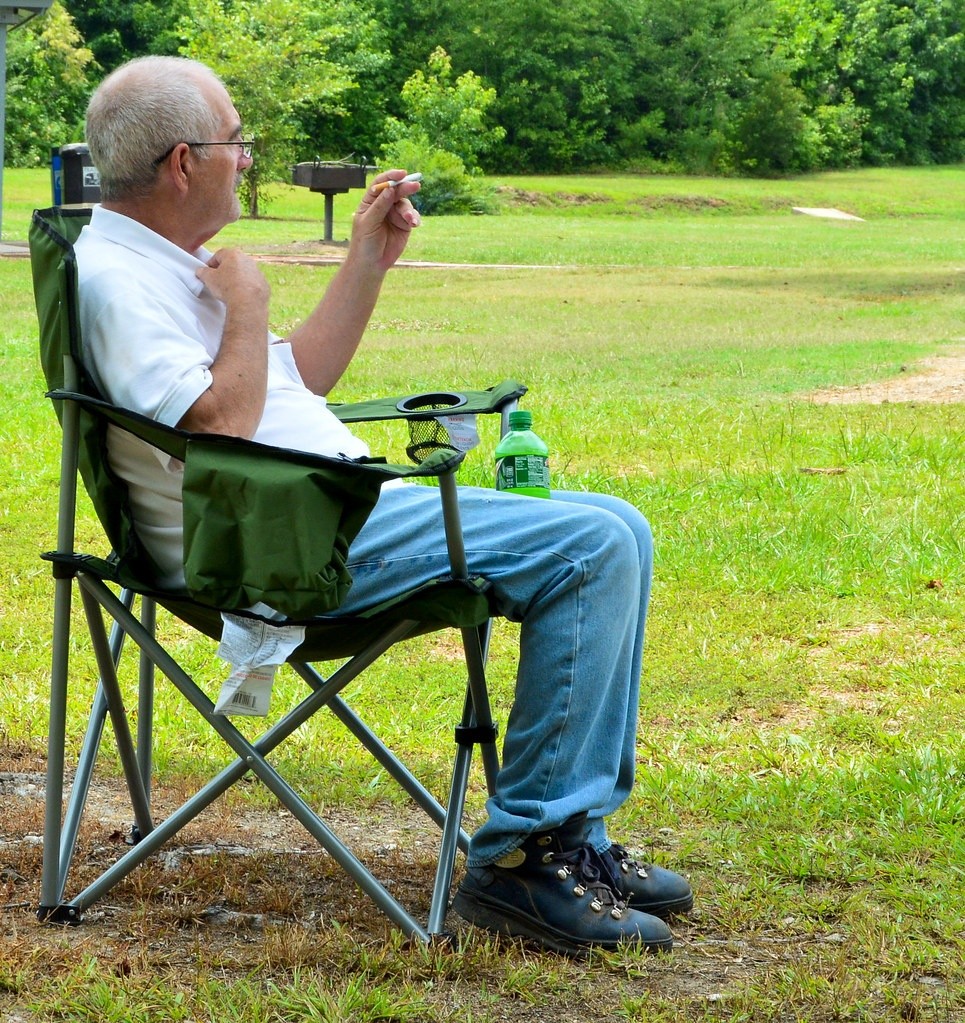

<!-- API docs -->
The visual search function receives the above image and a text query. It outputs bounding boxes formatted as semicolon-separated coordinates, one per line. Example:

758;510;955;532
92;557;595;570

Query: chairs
28;205;529;949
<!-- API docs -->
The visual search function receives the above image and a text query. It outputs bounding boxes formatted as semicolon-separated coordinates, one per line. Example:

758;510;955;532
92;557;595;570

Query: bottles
493;410;551;504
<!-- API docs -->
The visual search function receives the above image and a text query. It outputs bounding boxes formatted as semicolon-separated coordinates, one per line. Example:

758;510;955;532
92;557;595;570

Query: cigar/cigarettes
371;172;422;192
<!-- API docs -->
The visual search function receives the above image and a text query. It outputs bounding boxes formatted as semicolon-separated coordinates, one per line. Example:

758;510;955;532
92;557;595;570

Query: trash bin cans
51;145;63;206
63;144;102;203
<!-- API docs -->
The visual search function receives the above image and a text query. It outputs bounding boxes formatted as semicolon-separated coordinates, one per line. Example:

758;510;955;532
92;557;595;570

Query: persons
72;57;694;960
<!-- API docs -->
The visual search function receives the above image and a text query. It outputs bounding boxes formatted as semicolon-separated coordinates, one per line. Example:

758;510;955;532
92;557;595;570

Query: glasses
152;133;255;167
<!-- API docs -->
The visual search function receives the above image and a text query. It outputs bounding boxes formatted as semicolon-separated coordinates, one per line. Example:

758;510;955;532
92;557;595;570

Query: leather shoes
452;810;673;956
599;844;693;915
